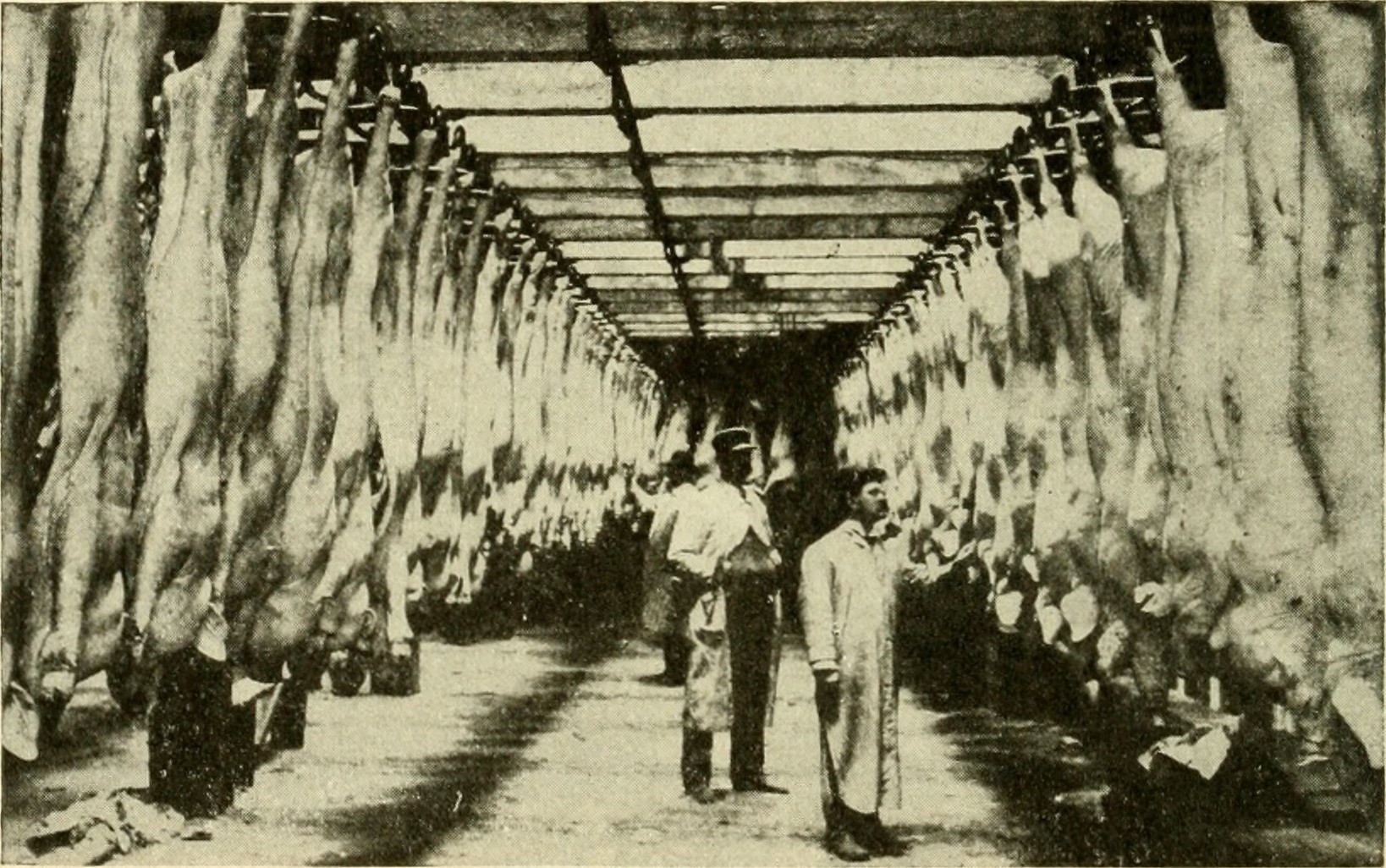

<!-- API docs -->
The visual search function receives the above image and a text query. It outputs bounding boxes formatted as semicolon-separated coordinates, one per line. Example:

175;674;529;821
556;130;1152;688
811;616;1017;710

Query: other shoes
689;784;716;803
829;833;867;860
735;777;788;793
640;672;680;688
873;826;905;856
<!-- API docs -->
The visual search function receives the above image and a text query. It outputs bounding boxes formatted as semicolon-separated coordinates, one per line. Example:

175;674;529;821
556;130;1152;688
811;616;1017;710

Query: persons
630;447;719;684
665;429;793;805
797;468;935;862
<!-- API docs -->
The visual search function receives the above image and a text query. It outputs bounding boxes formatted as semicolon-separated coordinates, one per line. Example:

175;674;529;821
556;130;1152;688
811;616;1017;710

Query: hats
712;427;757;455
661;451;704;478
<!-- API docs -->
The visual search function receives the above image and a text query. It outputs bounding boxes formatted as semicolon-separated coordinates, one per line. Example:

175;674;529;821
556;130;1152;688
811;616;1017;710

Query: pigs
656;392;802;504
830;0;1383;773
0;1;662;760
